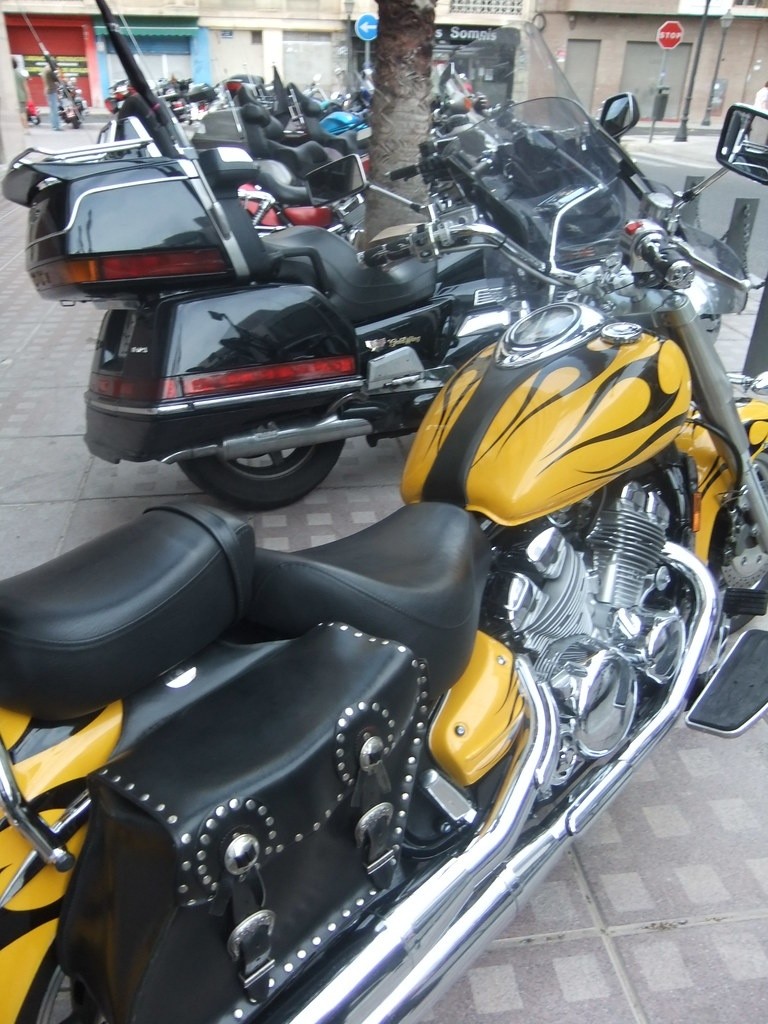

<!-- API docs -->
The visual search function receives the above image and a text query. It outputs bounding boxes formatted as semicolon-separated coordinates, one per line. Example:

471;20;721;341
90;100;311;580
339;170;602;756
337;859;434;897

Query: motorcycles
9;76;534;240
0;89;768;1024
2;18;730;511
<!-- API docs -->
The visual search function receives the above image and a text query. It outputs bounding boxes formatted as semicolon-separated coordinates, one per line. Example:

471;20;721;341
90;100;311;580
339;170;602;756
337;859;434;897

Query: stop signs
656;21;683;49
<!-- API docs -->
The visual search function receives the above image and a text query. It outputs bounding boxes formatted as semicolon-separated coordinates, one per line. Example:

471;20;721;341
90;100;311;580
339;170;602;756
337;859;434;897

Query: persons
754;80;768;111
10;58;33;136
43;63;67;131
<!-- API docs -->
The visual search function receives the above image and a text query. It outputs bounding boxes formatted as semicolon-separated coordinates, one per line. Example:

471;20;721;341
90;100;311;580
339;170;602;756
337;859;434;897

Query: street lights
701;8;734;126
